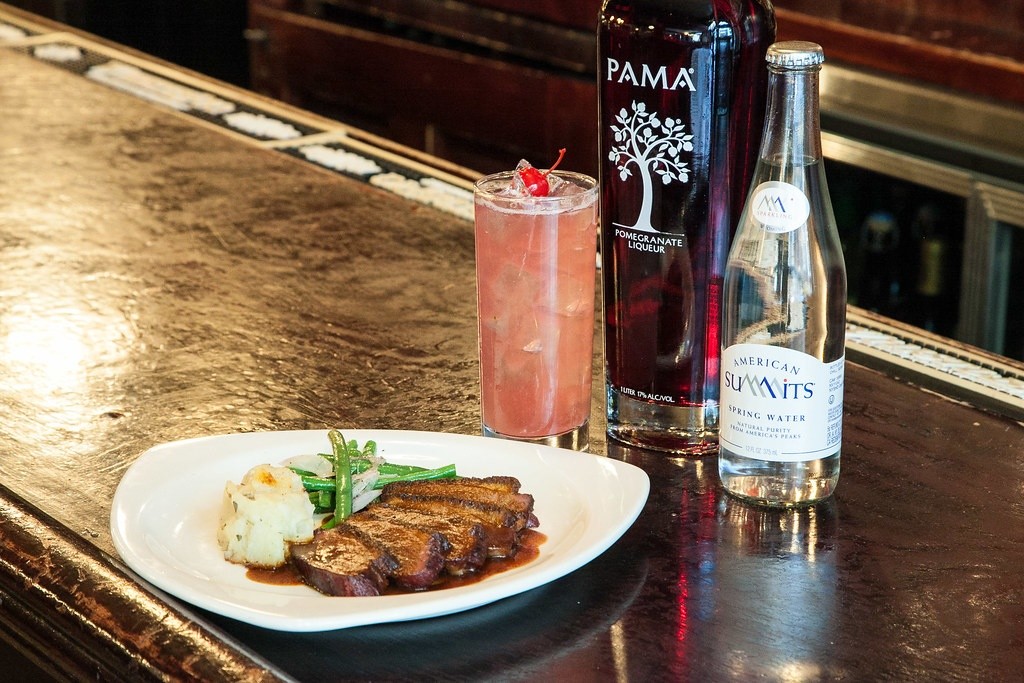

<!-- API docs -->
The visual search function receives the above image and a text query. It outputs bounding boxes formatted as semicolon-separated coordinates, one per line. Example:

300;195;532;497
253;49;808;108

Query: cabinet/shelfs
248;0;1024;360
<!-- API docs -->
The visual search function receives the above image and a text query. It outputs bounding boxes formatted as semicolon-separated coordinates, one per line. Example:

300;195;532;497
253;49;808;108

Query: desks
0;0;1024;683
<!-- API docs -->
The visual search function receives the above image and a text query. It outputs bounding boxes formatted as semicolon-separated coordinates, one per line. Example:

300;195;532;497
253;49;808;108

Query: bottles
592;0;780;457
717;39;846;508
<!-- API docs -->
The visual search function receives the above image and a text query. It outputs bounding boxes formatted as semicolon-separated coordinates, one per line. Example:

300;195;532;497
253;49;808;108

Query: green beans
279;429;457;531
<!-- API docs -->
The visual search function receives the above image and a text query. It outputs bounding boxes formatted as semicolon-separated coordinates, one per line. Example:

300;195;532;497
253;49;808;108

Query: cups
474;169;599;457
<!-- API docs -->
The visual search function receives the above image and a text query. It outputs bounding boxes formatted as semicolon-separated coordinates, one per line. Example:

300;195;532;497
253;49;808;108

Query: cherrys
512;148;566;197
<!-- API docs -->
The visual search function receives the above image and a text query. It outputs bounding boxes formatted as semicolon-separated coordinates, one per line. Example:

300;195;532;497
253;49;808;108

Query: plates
110;429;651;632
